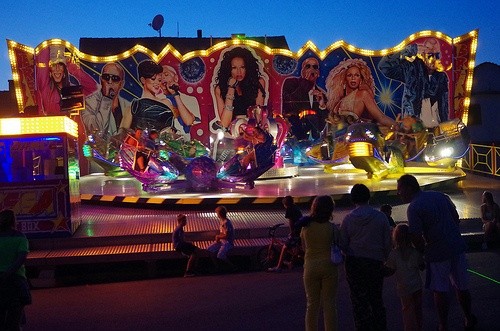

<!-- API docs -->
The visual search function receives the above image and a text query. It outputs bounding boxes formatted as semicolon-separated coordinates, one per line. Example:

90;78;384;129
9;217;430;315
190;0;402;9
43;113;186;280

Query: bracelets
225;94;235;101
223;104;234;111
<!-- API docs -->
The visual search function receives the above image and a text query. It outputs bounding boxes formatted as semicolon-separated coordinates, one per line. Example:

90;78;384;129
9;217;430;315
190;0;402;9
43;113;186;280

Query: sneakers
268;267;280;273
184;271;195;278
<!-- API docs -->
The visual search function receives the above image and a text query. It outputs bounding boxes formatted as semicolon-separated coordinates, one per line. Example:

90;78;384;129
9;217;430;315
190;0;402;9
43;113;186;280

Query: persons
477;189;499;252
203;206;235;276
378;44;448;136
334;182;393;331
109;59;177;151
324;58;395;138
297;194;341;331
267;195;307;273
172;214;205;279
380;204;397;276
396;174;476;331
214;46;266;132
279;58;329;147
80;62;136;140
45;46;81;114
158;64;203;140
384;220;427;331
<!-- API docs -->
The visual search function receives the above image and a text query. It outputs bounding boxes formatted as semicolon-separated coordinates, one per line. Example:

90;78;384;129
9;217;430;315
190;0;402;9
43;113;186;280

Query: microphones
163;82;179;90
109;88;115;98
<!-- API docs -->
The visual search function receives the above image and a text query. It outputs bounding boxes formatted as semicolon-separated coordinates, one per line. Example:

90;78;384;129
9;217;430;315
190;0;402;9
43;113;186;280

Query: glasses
426;52;441;60
101;74;121;82
305;64;319;70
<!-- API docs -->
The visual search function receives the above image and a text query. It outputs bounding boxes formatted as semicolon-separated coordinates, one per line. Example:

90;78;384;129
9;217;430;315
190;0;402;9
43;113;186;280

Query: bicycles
257;223;305;271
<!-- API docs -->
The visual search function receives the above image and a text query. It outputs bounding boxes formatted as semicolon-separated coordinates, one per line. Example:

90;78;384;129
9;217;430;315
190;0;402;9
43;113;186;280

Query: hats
49;44;67;66
